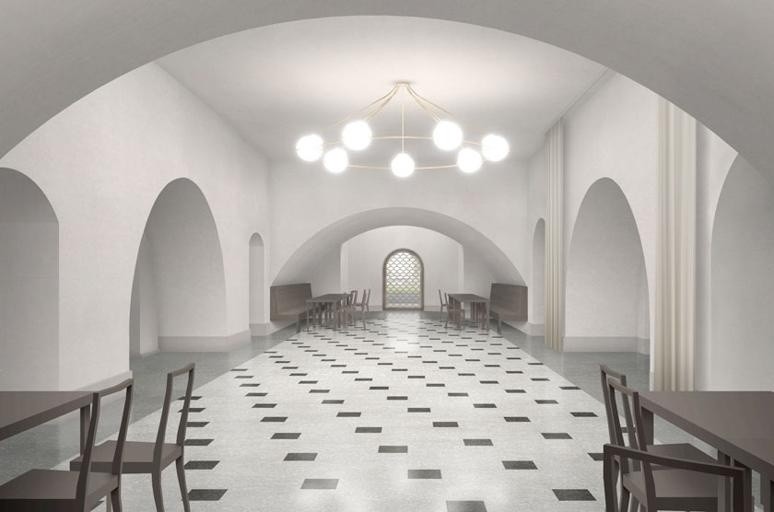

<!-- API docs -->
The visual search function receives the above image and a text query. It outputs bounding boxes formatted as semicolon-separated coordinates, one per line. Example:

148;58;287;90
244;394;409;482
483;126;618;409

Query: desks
448;294;490;332
0;391;91;456
637;391;774;512
306;294;353;332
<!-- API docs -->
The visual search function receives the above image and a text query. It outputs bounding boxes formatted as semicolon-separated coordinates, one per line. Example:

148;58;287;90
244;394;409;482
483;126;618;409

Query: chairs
70;362;195;512
0;378;134;512
337;289;371;330
439;289;465;330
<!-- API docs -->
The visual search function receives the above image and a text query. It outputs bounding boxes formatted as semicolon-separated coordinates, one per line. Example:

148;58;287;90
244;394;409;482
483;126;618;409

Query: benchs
270;282;326;334
476;283;528;334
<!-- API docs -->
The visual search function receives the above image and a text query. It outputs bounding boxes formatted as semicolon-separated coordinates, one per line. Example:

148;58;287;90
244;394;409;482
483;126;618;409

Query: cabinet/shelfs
600;364;752;512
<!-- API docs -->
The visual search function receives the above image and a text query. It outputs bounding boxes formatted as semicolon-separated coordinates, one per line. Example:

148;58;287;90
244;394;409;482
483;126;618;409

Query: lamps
294;80;510;180
